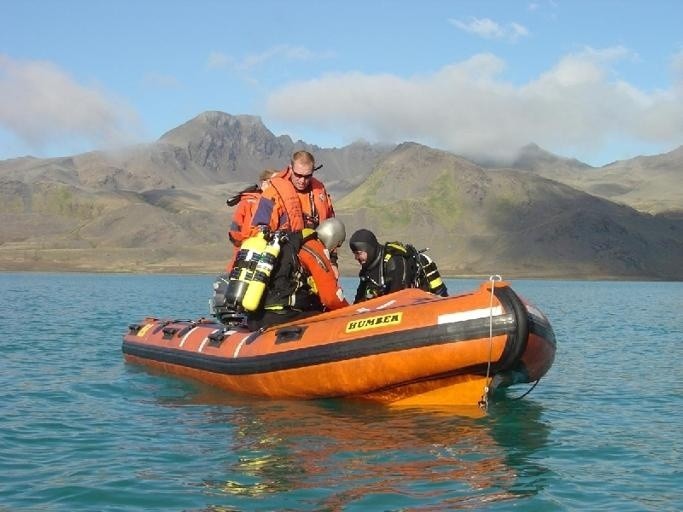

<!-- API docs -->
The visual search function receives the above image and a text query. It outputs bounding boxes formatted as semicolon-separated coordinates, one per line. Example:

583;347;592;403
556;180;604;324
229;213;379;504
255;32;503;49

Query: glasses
292;166;312;178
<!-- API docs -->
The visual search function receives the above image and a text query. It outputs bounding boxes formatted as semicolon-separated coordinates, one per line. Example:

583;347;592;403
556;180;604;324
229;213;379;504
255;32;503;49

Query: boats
121;275;557;407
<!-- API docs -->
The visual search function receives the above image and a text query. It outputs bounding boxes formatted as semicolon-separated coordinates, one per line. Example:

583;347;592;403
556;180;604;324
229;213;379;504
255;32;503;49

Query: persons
225;168;283;276
247;149;340;288
348;229;451;304
244;216;354;333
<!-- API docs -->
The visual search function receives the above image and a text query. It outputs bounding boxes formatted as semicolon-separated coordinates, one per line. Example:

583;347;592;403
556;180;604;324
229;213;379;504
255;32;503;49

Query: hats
349;229;378;254
315;217;346;248
